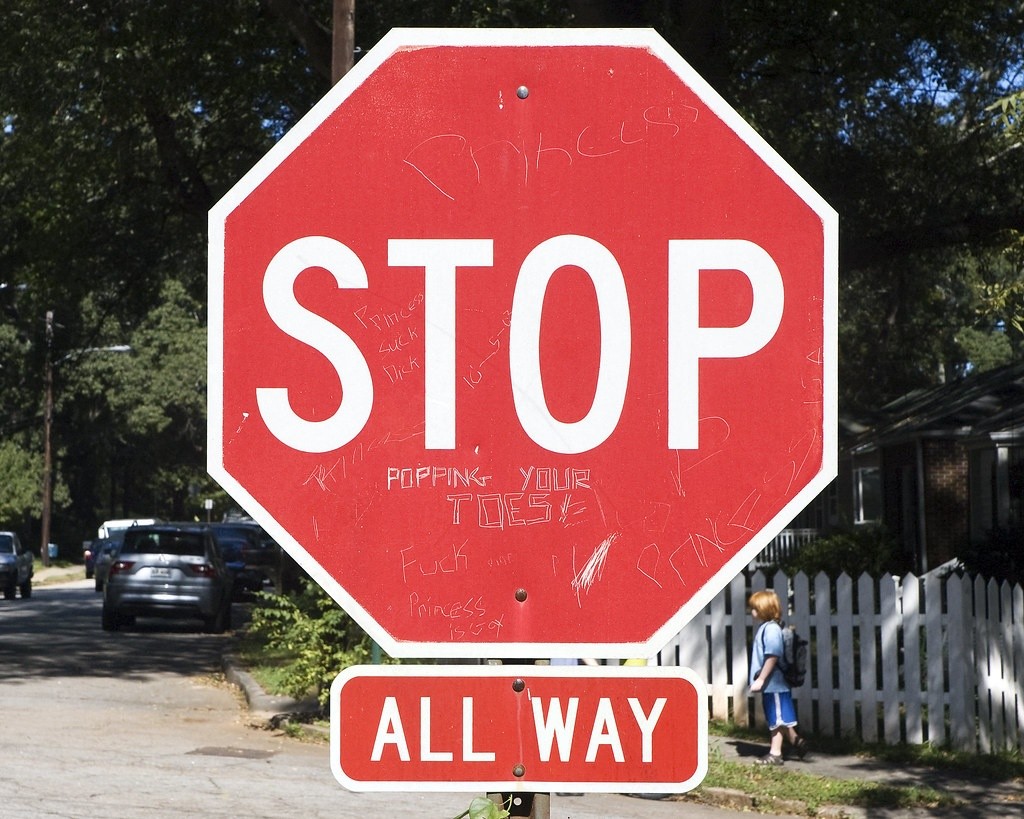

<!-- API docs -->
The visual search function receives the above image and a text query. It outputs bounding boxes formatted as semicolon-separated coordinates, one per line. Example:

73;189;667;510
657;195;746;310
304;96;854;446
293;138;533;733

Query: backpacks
762;625;809;687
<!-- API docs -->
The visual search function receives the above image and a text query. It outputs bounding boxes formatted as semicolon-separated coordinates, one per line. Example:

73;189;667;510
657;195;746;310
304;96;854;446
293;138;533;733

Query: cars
103;520;233;634
81;507;287;594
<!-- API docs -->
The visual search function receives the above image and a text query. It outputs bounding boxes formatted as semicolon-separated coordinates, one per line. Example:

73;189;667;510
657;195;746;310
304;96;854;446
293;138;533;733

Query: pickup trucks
0;531;39;599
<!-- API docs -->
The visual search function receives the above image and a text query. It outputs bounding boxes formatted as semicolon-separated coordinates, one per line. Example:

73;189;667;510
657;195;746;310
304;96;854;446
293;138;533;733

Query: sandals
793;734;806;760
754;754;784;766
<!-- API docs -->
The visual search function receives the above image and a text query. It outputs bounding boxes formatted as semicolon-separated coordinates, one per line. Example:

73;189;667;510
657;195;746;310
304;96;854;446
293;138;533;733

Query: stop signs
206;26;842;662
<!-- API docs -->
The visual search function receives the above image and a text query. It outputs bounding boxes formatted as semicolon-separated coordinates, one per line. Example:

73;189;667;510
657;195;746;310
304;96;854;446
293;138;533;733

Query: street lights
37;311;133;564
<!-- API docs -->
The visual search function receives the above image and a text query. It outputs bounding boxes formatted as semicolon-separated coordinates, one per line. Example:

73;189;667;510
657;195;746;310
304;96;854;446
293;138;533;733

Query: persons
749;590;810;765
